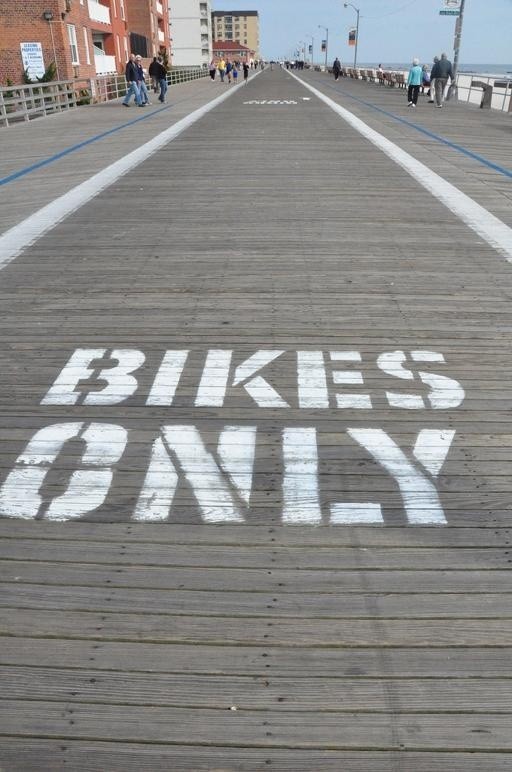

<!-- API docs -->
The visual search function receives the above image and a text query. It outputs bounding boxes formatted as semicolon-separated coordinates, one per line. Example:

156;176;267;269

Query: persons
428;56;440;104
209;58;264;83
378;64;386;78
149;57;159;94
406;58;423;107
431;53;454;108
422;65;431;96
156;57;168;103
280;60;304;70
122;53;152;108
333;58;341;81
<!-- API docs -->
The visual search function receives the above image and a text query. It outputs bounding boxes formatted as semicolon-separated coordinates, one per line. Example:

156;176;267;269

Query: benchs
341;68;430;93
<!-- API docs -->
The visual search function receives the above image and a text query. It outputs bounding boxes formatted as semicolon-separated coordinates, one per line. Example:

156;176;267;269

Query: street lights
271;34;314;65
344;2;360;70
317;25;328;67
